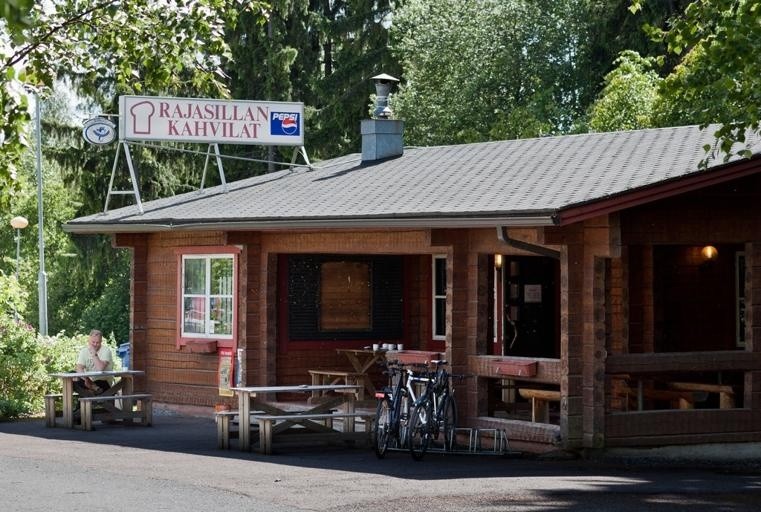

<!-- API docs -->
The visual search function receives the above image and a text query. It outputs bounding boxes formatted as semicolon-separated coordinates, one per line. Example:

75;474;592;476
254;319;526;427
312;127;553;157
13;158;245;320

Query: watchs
92;353;99;358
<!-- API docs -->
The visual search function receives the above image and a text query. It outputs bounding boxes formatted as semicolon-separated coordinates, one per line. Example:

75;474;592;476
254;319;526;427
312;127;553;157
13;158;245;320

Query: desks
49;371;146;428
335;348;388;399
230;385;365;452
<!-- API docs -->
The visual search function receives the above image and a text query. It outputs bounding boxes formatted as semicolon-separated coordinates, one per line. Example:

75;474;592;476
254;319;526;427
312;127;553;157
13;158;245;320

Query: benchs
254;414;378;455
518;388;561;424
308;369;367;409
78;394;151;430
43;394;77;427
216;410;338;449
671;381;737;410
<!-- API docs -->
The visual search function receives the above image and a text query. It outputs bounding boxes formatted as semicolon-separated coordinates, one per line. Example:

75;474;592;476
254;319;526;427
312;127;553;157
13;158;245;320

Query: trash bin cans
116;343;130;370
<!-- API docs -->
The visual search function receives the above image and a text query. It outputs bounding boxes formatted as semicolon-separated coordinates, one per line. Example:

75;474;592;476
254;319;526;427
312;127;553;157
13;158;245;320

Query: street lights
25;83;52;337
10;217;28;281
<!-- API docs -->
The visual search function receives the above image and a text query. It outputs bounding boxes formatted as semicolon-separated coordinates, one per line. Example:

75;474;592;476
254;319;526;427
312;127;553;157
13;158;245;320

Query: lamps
494;253;502;272
701;242;719;263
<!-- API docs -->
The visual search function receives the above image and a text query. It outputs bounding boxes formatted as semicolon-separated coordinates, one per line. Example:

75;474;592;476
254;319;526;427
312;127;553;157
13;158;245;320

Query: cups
372;343;379;351
382;343;396;351
397;343;404;350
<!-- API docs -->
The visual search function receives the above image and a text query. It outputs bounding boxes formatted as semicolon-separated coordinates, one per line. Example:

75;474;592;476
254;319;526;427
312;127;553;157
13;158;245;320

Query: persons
69;329;114;414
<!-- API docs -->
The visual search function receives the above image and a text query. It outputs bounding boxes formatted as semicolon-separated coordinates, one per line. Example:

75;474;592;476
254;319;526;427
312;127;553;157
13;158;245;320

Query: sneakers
73;388;92;415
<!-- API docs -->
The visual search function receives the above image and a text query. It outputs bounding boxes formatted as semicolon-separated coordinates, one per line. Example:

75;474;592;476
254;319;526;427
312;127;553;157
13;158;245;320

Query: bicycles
372;357;476;463
